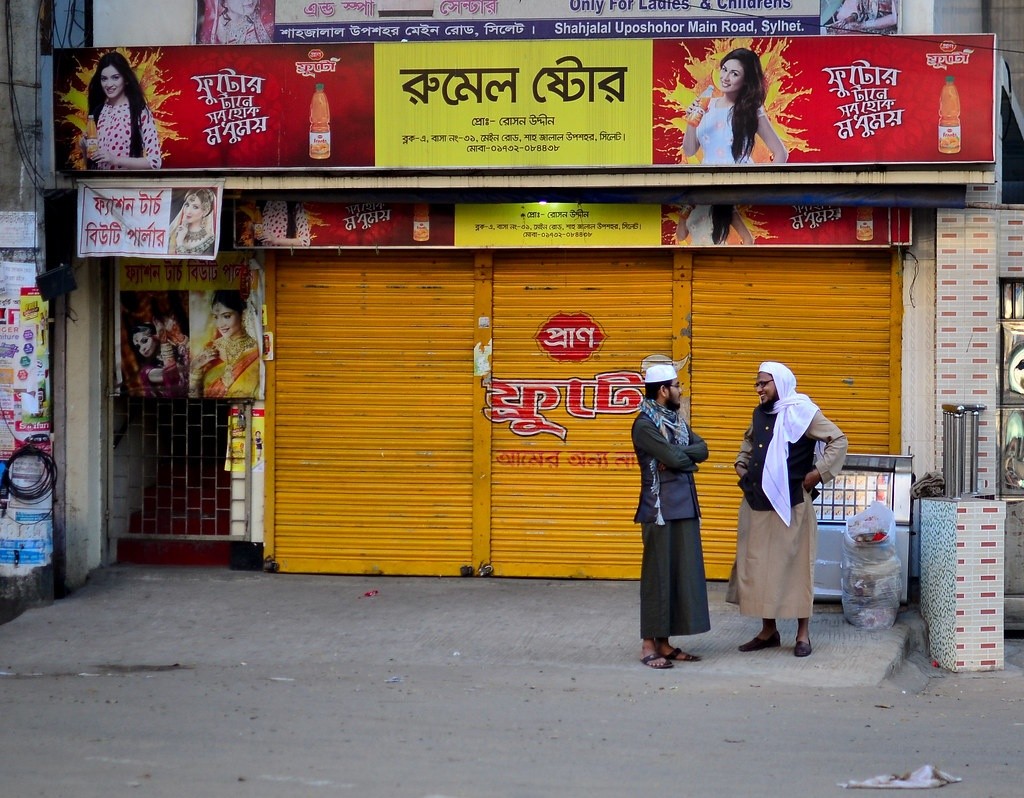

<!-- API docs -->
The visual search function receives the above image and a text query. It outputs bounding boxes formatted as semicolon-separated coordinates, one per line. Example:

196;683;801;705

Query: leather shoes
738;630;780;651
795;635;812;656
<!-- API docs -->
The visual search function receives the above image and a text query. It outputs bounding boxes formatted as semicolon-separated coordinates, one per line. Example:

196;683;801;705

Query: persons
186;290;260;398
683;48;788;165
199;0;273;44
676;204;754;244
630;364;710;667
132;315;189;396
168;188;217;255
724;362;848;657
79;52;162;170
255;201;310;246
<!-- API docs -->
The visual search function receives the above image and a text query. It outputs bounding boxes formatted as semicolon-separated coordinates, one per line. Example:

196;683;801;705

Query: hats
645;364;677;383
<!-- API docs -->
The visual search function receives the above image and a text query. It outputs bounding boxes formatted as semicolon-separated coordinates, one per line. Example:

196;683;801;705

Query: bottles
254;207;262;238
682;205;693;220
938;75;961;154
309;83;330;159
413;197;429;241
685;85;716;127
86;115;98;158
856;207;873;241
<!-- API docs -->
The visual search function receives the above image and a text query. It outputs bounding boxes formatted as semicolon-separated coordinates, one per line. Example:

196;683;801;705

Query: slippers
664;648;701;661
640;653;673;668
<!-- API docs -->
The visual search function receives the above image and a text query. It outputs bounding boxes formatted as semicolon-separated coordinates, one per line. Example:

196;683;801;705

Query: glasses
754;379;774;388
670;382;679;388
